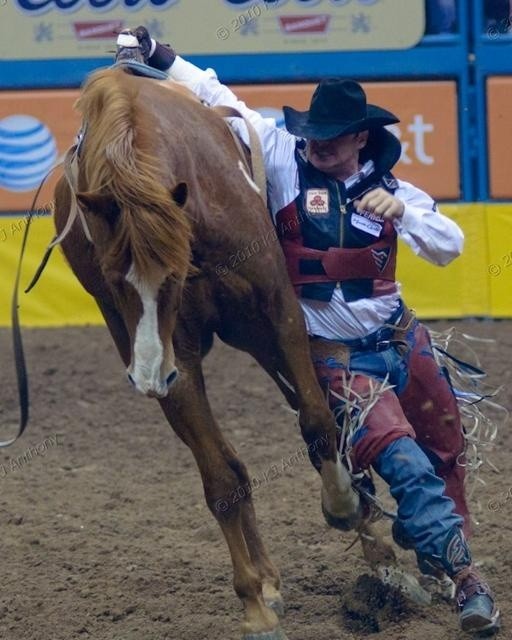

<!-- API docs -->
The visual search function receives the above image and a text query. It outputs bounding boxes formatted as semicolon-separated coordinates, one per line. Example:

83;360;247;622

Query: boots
418;553;457;604
432;529;500;636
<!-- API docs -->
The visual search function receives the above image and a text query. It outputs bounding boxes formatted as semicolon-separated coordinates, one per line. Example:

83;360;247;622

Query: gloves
119;25;175;70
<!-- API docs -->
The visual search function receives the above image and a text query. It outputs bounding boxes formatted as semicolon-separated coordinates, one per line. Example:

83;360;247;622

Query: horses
54;65;398;640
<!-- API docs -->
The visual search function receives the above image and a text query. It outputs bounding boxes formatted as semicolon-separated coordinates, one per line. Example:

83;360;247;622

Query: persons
117;25;503;635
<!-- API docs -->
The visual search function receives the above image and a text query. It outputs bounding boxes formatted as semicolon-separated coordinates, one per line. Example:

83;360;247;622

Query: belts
353;326;407;352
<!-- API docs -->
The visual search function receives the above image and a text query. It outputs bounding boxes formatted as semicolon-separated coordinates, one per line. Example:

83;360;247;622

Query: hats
282;77;401;141
